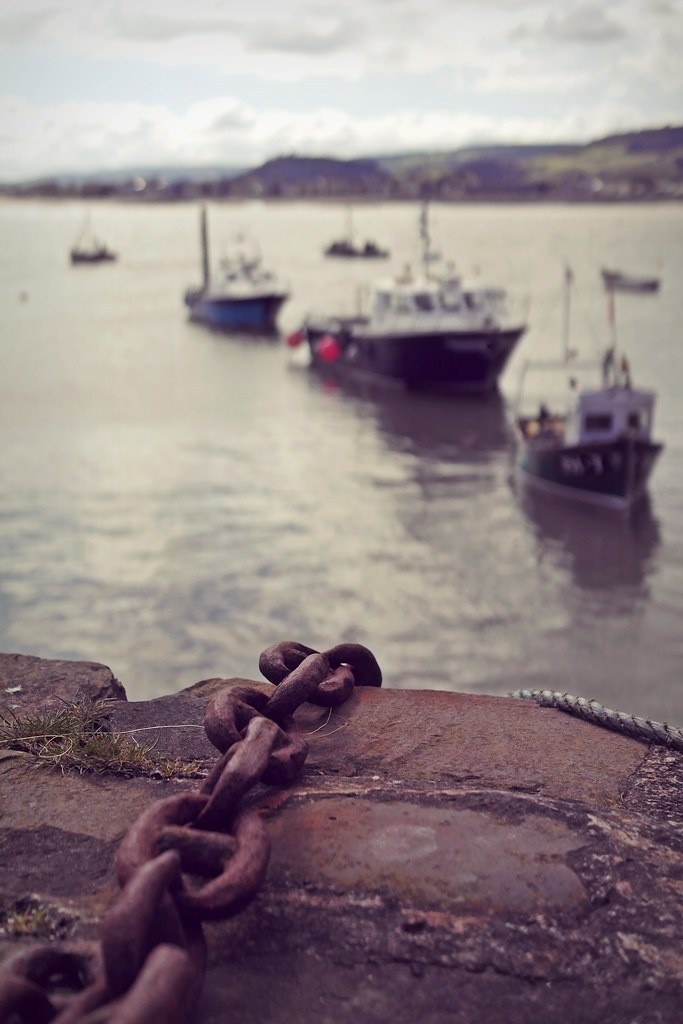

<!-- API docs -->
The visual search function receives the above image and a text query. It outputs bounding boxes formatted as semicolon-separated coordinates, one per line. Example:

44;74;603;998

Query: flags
600;268;660;295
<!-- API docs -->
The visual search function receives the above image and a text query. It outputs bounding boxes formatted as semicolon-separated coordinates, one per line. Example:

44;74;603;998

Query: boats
325;236;388;266
289;189;529;395
510;260;670;516
182;194;290;334
70;231;116;264
